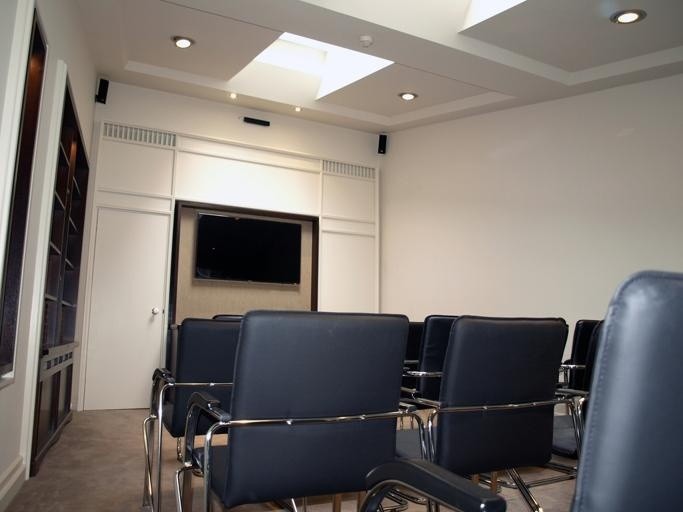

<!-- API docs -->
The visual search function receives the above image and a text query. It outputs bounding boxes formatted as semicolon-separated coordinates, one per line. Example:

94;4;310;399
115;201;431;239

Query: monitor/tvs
192;213;302;287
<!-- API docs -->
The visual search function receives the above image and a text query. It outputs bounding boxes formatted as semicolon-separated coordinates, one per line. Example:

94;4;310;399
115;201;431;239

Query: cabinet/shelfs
29;72;89;478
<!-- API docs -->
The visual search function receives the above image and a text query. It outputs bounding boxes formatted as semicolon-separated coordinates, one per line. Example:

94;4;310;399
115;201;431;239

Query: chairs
340;271;682;510
137;313;608;512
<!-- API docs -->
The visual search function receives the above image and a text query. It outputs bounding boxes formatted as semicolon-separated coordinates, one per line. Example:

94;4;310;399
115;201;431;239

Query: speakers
244;117;270;126
95;78;109;104
378;134;387;153
